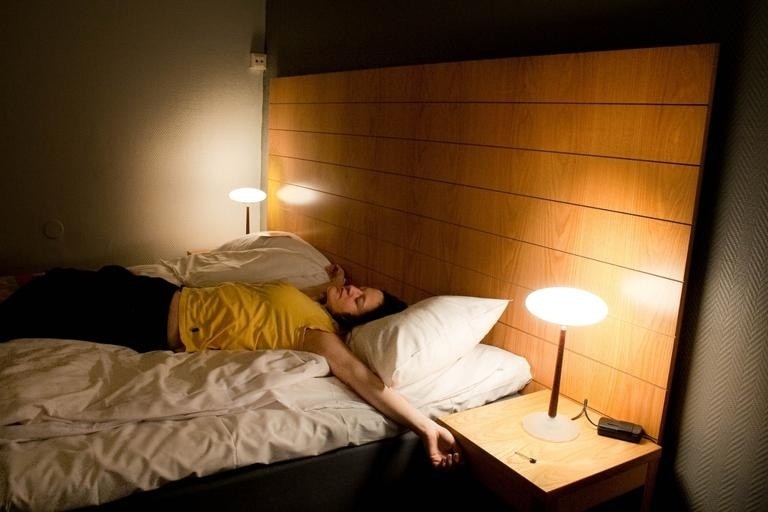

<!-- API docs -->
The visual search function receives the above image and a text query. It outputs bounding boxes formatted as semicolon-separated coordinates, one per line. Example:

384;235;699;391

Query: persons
0;262;463;468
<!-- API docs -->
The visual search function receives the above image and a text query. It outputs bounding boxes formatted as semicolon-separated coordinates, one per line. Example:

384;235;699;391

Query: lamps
519;286;610;443
228;187;268;235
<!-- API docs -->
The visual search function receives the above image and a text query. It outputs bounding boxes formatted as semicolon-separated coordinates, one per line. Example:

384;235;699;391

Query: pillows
213;230;346;291
345;293;514;392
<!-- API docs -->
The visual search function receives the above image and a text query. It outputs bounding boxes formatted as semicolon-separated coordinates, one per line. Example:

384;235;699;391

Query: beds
0;263;533;511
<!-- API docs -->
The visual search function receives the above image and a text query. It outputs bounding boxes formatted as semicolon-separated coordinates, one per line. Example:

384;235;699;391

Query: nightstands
435;386;663;512
187;248;210;256
